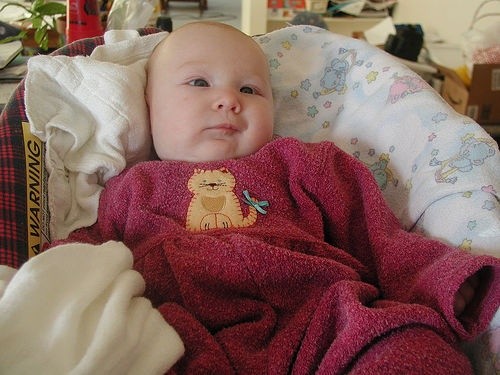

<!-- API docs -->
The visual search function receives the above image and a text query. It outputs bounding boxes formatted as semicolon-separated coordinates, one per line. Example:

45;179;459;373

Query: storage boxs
441;63;500;126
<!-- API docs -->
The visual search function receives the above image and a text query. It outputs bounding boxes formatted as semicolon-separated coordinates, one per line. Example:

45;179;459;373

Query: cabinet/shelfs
267;17;391;51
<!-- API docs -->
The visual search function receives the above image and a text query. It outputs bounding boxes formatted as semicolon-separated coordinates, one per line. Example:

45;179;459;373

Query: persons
41;21;500;375
